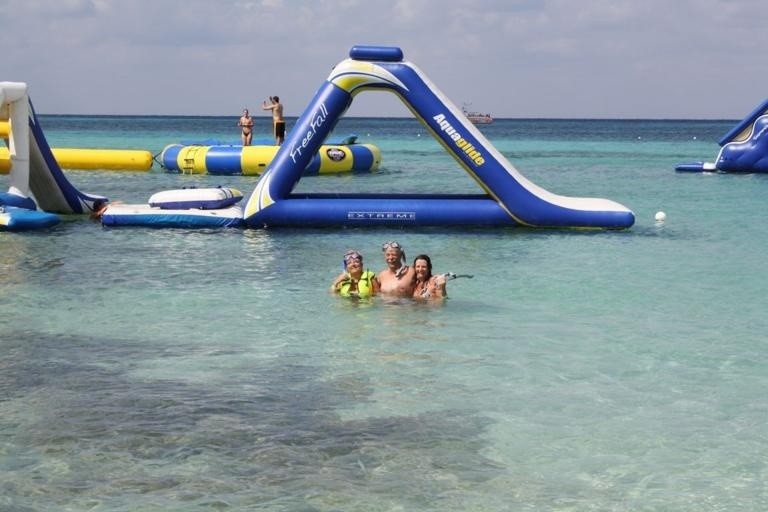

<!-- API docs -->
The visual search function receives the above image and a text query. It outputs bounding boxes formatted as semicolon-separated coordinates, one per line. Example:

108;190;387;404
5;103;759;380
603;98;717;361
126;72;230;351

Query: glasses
383;242;399;249
344;252;361;261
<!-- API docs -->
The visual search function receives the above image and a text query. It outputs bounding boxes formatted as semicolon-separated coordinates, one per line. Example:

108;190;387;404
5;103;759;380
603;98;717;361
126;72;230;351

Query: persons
331;250;379;298
90;200;107;220
263;96;285;145
412;255;446;299
377;242;416;298
240;109;253;146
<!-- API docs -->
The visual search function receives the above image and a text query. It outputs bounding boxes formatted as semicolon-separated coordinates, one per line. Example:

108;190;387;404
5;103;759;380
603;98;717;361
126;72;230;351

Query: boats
160;135;380;176
460;101;493;125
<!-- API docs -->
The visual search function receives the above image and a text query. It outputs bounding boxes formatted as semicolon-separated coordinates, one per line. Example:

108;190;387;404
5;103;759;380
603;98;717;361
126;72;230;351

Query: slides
0;81;108;213
400;63;635;229
674;98;767;173
